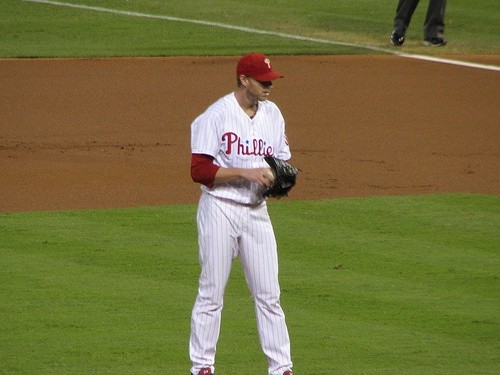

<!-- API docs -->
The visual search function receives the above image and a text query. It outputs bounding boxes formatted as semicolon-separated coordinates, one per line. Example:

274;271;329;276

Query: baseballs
263;172;275;186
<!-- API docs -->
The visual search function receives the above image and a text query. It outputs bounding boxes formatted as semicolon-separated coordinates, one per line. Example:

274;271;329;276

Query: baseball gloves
261;156;296;199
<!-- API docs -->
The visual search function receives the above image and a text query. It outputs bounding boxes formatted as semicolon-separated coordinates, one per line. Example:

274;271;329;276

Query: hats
236;52;285;82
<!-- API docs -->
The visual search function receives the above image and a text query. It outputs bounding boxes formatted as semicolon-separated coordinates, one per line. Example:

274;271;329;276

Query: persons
389;0;448;48
187;52;297;375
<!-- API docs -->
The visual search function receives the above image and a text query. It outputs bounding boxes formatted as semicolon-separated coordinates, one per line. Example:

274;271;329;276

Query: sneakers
422;37;448;48
390;29;405;48
